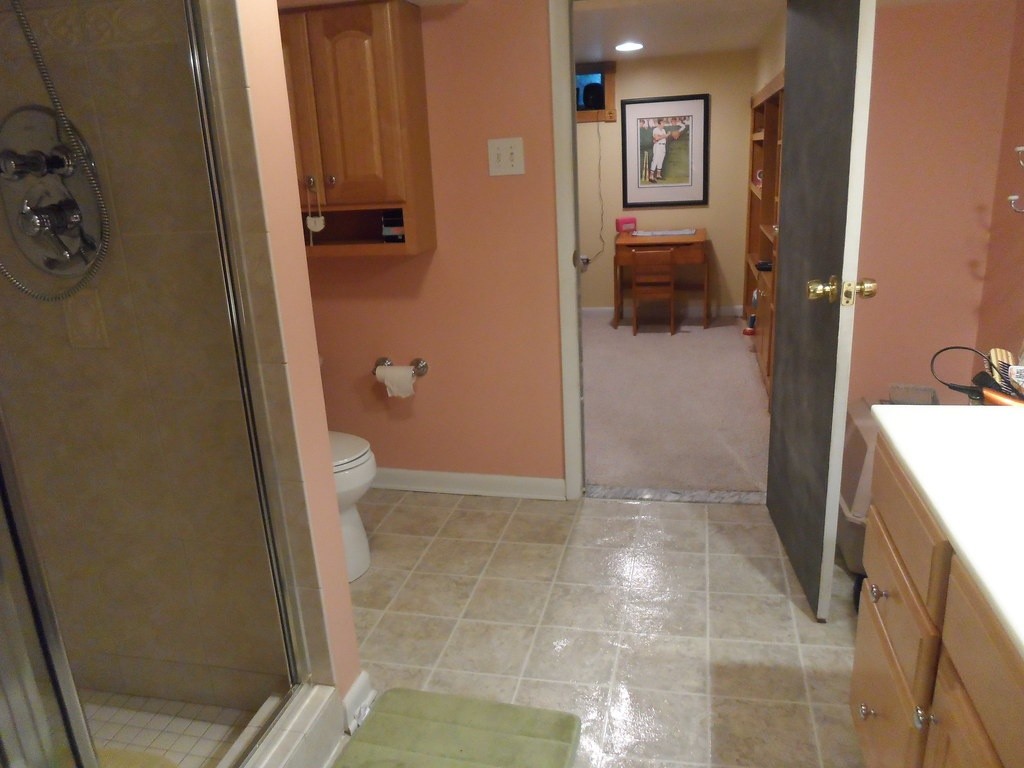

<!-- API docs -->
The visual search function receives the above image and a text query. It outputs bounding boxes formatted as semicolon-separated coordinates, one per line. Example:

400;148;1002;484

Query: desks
613;227;711;329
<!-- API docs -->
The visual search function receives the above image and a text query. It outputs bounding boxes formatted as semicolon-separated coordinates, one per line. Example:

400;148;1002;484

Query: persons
639;117;689;183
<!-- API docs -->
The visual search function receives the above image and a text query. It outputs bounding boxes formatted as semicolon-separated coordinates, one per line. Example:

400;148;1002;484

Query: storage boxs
838;402;876;577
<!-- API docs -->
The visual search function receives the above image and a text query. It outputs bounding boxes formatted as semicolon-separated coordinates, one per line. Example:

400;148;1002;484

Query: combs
983;348;1015;394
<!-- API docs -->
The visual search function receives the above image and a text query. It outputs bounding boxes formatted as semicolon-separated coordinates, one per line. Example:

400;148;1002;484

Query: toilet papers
375;365;417;398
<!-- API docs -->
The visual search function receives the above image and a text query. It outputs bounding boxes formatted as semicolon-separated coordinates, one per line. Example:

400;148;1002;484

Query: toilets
327;431;378;583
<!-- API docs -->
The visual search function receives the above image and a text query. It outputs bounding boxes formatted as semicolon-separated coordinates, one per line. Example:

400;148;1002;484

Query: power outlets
487;136;526;177
579;255;590;268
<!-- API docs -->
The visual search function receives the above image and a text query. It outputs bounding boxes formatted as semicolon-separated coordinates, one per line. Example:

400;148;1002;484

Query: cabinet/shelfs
278;0;437;258
741;85;784;400
848;429;1024;768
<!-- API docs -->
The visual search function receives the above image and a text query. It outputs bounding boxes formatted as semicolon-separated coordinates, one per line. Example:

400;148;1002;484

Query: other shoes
649;178;657;184
656;176;665;180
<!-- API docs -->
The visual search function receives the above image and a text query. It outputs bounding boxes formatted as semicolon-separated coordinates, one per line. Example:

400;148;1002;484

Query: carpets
331;688;581;768
54;744;178;768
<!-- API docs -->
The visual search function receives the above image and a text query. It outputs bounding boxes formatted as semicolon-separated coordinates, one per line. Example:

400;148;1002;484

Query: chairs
631;247;678;336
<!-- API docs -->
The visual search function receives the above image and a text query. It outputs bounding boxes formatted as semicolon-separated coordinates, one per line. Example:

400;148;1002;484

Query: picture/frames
620;93;709;209
